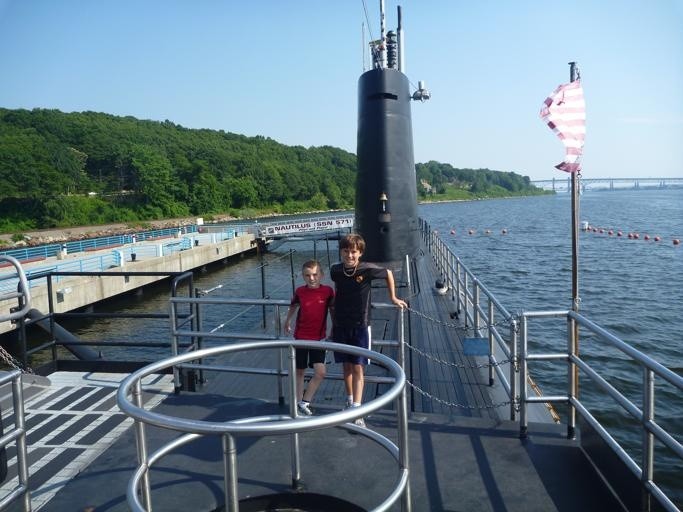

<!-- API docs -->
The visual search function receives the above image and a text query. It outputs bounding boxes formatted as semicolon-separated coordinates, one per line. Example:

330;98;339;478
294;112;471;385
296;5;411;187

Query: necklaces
342;261;357;277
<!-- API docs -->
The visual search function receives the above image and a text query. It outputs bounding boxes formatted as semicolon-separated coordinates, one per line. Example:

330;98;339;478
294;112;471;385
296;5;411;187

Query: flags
540;80;586;172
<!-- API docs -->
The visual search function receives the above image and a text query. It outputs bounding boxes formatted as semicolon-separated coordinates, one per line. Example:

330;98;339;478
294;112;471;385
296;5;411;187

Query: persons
284;261;335;416
330;234;408;426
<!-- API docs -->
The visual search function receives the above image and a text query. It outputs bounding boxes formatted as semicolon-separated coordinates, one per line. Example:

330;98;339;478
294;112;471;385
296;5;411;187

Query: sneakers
343;401;367;428
297;401;313;415
